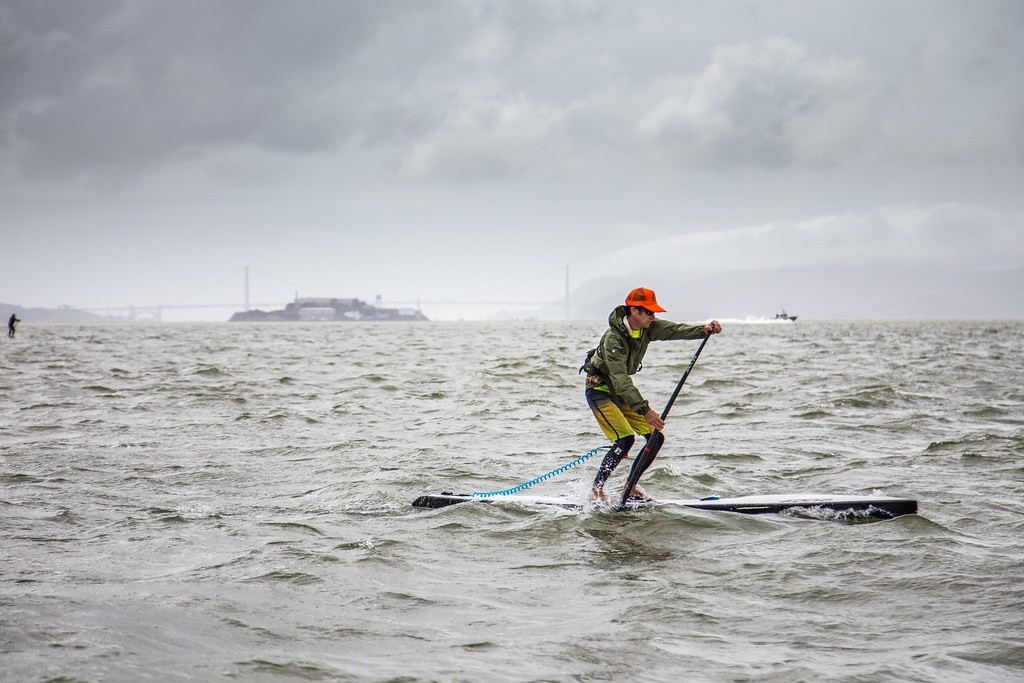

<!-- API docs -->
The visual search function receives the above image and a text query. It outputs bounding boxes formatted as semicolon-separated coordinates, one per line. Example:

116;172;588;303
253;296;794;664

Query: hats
625;287;667;312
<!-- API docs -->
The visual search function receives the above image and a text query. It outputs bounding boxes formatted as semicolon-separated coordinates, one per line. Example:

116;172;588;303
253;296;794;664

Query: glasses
636;307;654;315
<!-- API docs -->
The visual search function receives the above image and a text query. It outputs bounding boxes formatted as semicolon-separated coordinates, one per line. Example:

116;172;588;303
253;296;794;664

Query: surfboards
415;493;925;526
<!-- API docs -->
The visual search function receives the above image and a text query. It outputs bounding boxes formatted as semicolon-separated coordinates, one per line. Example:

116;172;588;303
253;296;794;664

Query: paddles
615;327;717;505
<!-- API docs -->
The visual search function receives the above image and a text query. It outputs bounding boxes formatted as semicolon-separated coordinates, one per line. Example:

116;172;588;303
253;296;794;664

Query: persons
8;314;20;339
585;287;722;506
